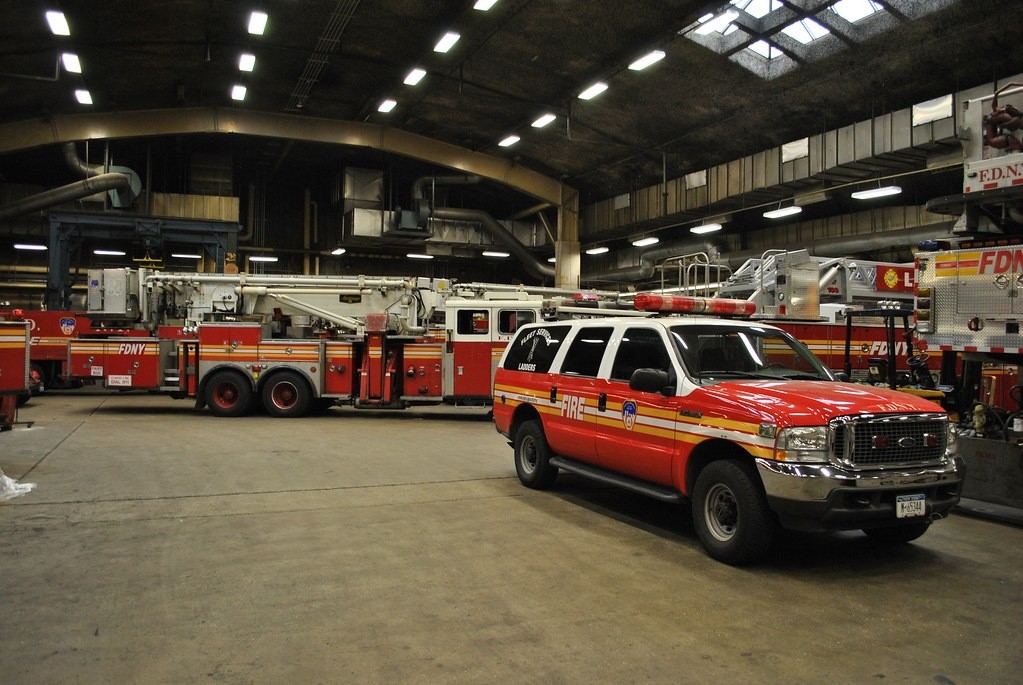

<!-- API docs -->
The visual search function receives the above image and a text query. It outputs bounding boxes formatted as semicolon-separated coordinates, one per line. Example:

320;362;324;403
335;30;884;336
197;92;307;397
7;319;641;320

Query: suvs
492;291;964;551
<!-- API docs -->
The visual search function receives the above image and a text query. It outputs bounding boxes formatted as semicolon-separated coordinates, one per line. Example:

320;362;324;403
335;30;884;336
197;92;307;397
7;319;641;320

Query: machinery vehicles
0;239;1023;508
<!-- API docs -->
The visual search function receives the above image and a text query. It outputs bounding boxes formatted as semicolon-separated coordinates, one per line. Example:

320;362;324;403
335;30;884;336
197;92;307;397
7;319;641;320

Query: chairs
866;357;911;387
